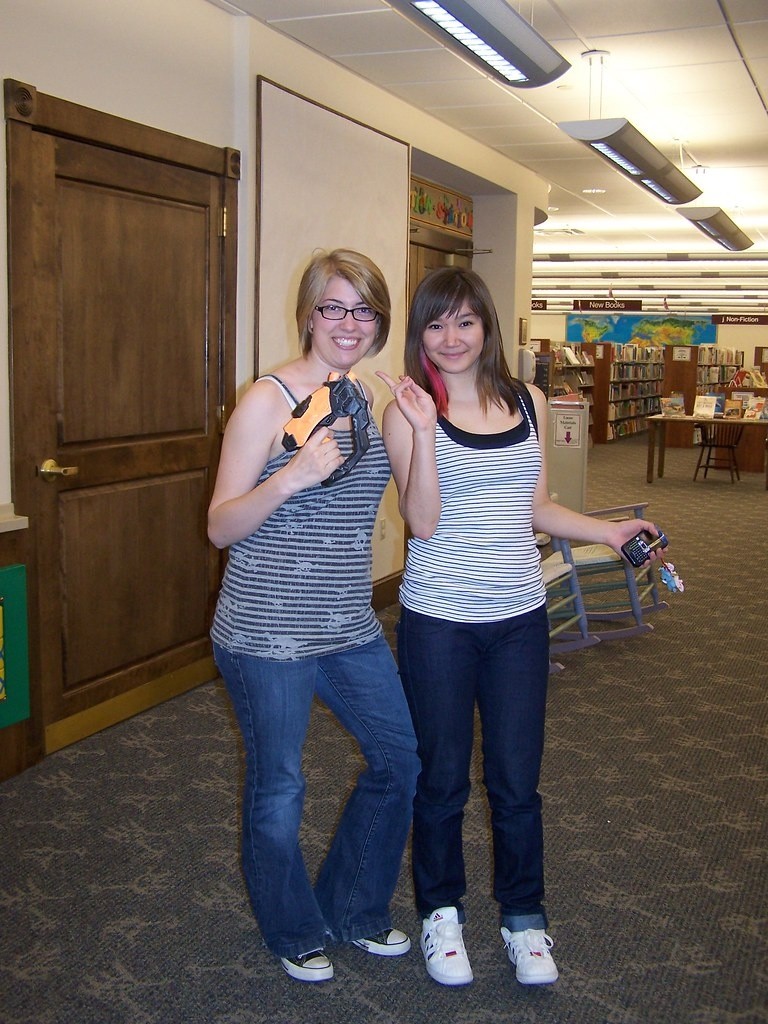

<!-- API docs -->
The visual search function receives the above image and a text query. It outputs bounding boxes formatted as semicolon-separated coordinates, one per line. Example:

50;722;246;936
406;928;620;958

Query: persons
372;266;660;987
205;246;425;983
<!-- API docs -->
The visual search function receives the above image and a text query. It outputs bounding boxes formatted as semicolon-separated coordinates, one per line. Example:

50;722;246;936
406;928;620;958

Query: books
548;338;768;441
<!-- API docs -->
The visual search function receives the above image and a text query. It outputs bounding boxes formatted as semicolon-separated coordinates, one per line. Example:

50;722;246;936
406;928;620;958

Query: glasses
314;304;379;323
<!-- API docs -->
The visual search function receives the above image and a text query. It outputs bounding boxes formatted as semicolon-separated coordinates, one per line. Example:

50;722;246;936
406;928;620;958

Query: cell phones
622;525;668;566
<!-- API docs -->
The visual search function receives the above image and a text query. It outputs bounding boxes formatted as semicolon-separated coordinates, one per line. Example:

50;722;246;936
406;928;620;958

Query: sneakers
499;926;559;984
278;951;335;981
418;906;473;985
350;927;411;956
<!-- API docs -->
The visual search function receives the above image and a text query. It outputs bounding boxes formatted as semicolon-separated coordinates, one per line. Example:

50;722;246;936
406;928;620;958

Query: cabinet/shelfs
532;336;745;443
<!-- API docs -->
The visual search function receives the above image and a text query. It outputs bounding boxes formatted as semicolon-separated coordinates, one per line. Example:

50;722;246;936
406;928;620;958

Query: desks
646;406;768;494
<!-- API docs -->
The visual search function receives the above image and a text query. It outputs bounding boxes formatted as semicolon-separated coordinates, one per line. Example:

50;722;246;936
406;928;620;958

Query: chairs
549;491;671;638
691;419;743;485
533;535;601;675
